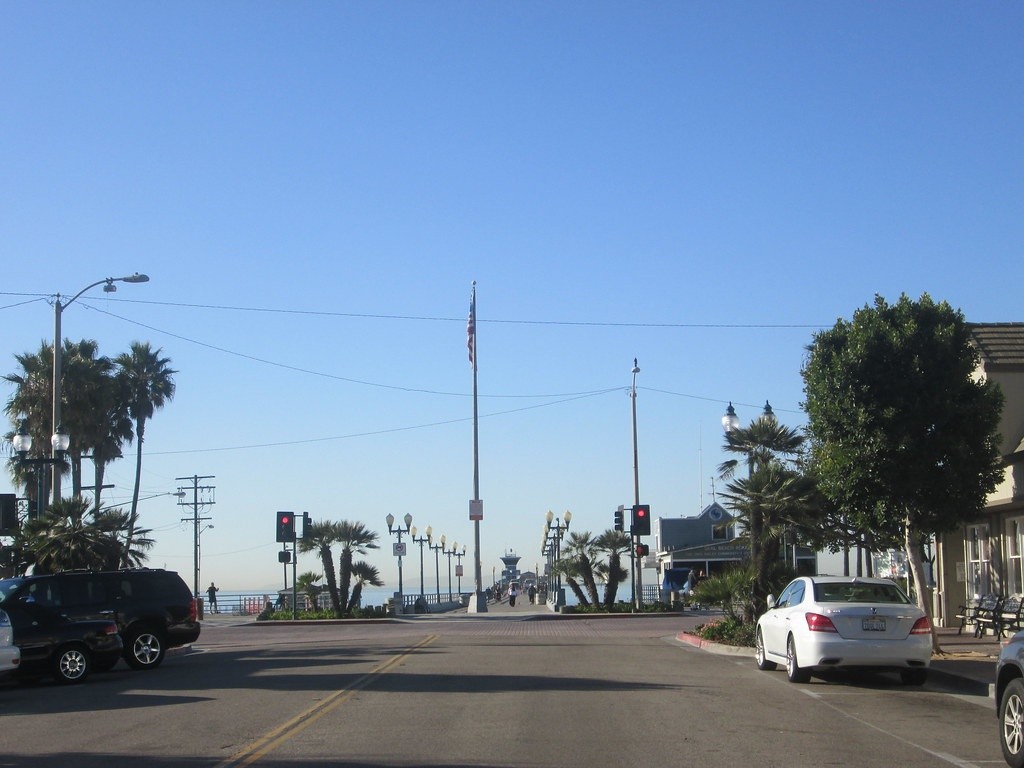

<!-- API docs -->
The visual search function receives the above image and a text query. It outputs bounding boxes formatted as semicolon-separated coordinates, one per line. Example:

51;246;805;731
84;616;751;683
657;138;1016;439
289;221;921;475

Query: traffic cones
51;270;154;509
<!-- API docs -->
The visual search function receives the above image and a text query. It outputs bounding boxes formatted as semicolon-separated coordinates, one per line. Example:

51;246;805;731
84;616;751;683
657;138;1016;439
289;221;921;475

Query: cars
0;609;21;680
992;626;1024;768
0;593;124;685
753;574;934;684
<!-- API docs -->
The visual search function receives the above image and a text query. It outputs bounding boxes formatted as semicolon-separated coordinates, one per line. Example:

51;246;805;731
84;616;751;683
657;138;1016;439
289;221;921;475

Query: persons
687;570;707;591
508;583;517;607
415;596;430;613
486;586;490;594
496;585;503;600
206;582;219;615
528;583;536;604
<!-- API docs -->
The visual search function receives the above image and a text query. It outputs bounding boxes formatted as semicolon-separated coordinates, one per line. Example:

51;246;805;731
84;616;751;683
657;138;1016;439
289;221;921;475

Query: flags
467;293;475;362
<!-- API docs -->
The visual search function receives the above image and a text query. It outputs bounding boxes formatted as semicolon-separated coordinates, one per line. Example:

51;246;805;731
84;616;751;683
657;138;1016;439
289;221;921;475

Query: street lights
721;400;780;623
192;523;214;600
628;358;643;612
539;510;573;607
409;524;433;596
441;540;458;603
9;428;71;524
385;512;413;596
450;545;467;594
429;535;447;603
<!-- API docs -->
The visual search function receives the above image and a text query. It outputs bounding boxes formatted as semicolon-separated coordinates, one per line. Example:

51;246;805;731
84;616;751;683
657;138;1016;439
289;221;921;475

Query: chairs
957;593;1024;641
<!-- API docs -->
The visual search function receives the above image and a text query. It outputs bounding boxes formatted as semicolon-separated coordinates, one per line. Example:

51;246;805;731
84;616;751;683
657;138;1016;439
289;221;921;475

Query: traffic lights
278;552;291;563
615;505;624;535
275;511;295;543
638;545;649;556
303;512;313;538
632;504;651;535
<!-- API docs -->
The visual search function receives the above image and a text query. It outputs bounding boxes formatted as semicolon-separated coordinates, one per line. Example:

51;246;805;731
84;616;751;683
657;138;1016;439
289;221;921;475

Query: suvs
0;566;201;674
